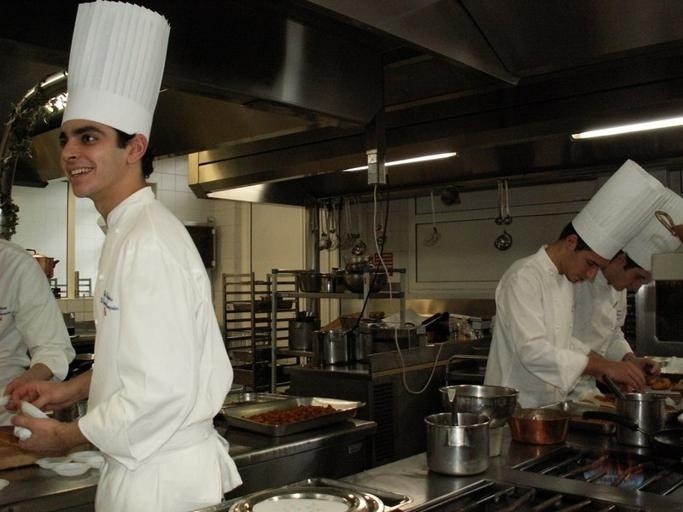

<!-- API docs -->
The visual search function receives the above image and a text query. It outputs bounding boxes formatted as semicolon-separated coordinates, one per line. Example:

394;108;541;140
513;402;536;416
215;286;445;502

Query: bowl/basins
296;273;344;293
509;409;571;446
341;274;388;294
439;384;518;427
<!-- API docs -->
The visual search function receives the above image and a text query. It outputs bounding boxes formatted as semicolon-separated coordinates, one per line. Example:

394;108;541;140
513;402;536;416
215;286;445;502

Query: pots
288;320;320;349
26;248;59;279
616;393;665;446
426;413;489;476
313;330;351;366
582;412;682;454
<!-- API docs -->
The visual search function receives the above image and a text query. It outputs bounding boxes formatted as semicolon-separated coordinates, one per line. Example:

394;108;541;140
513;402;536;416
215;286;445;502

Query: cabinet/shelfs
220;272;305;392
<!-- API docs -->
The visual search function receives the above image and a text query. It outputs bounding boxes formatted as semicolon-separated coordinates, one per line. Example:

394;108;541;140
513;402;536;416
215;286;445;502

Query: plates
19;400;50;420
36;447;104;479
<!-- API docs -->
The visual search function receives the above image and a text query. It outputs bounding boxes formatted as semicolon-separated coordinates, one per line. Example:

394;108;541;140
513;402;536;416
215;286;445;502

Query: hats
570;157;667;262
618;187;683;274
58;1;172;145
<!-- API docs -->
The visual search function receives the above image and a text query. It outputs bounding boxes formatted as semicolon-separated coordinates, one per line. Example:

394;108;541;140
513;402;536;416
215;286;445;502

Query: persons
481;219;647;413
616;273;653;327
5;118;244;511
0;235;77;429
562;249;660;397
